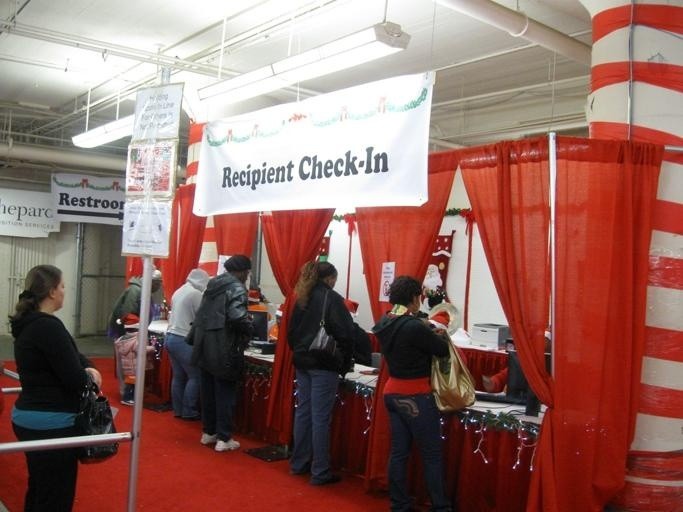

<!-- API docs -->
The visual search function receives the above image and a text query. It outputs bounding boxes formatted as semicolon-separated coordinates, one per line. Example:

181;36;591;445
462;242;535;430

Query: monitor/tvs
504;349;552;418
247;310;269;342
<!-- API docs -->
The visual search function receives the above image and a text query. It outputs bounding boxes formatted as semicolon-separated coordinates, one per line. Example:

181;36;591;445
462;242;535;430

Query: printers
471;322;511;351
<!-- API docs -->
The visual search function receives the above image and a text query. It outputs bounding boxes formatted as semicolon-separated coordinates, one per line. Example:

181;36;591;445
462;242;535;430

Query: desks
146;320;546;510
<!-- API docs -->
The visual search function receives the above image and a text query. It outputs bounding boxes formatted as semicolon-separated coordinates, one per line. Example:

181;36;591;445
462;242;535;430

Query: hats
224;254;252;271
116;313;140;329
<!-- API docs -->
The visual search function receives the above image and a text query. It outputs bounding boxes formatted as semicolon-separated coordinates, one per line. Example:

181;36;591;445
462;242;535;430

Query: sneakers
182;415;201;420
296;463;340;485
201;432;240;452
120;400;134;406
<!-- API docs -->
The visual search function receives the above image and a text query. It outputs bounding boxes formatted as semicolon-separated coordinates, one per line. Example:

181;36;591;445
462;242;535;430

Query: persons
243;288;272;322
11;264;103;511
109;266;163;400
114;314;155;407
186;255;256;451
269;304;285;342
372;275;453;512
343;297;371;365
481;325;553;393
288;262;355;486
428;311;468;367
164;269;209;419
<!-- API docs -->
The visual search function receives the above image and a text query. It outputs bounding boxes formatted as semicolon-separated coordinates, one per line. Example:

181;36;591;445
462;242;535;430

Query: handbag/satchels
76;374;118;464
185;322;195;345
429;328;476;412
124;375;135;385
308;327;350;374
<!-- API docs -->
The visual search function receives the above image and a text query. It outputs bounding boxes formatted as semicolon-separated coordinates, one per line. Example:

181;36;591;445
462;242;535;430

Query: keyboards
474;393;527;405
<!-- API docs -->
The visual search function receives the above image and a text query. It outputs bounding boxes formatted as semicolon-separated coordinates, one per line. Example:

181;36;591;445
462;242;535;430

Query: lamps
195;5;412;123
72;52;182;149
